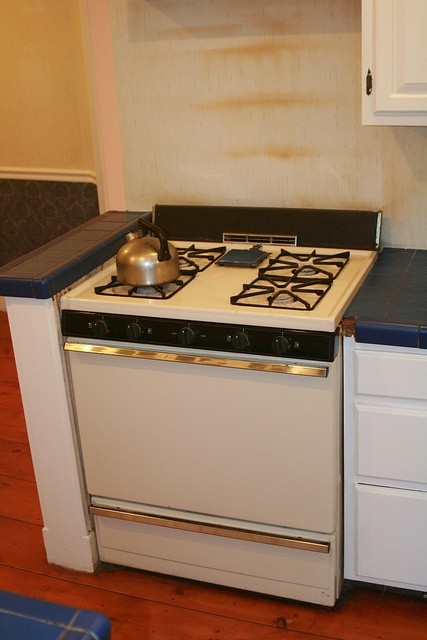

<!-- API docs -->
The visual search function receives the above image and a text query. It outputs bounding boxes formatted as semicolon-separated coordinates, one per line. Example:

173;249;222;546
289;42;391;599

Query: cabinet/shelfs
360;0;427;128
343;322;427;590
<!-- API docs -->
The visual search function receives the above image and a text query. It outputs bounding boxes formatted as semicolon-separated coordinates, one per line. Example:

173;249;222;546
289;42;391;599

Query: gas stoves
59;204;385;363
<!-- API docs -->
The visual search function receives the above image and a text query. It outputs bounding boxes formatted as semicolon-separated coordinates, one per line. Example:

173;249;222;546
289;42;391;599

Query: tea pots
114;216;182;287
